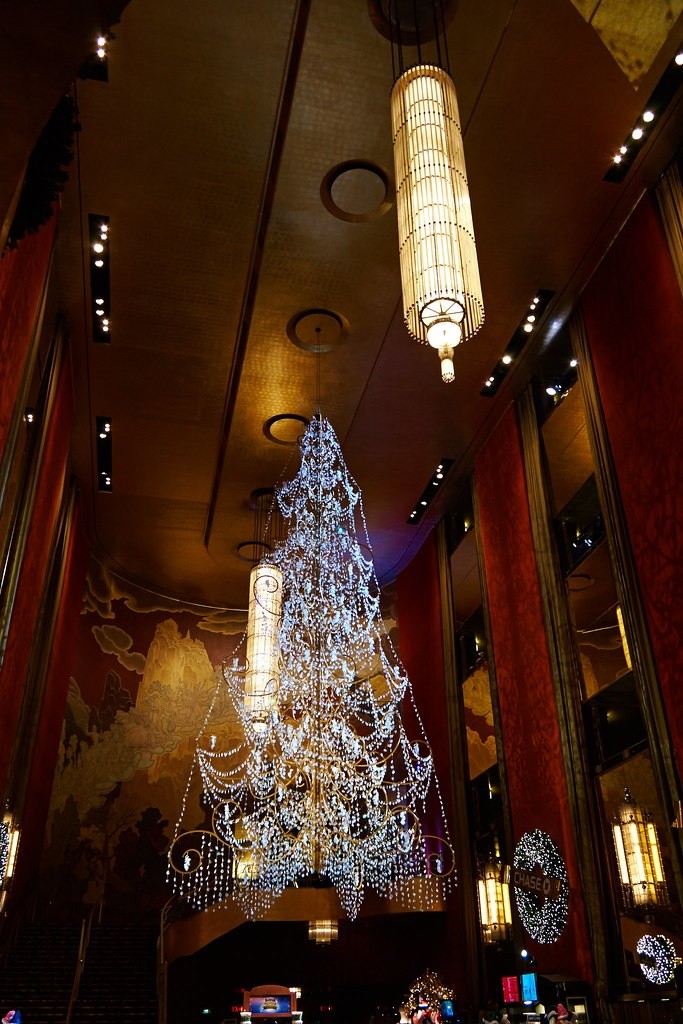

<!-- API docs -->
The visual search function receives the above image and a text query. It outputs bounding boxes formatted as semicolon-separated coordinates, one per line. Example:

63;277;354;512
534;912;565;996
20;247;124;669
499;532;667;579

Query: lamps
165;328;461;923
610;765;672;925
368;0;486;385
244;488;284;734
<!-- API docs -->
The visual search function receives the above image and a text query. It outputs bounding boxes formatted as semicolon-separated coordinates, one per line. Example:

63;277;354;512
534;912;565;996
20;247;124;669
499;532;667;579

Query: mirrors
475;833;513;945
